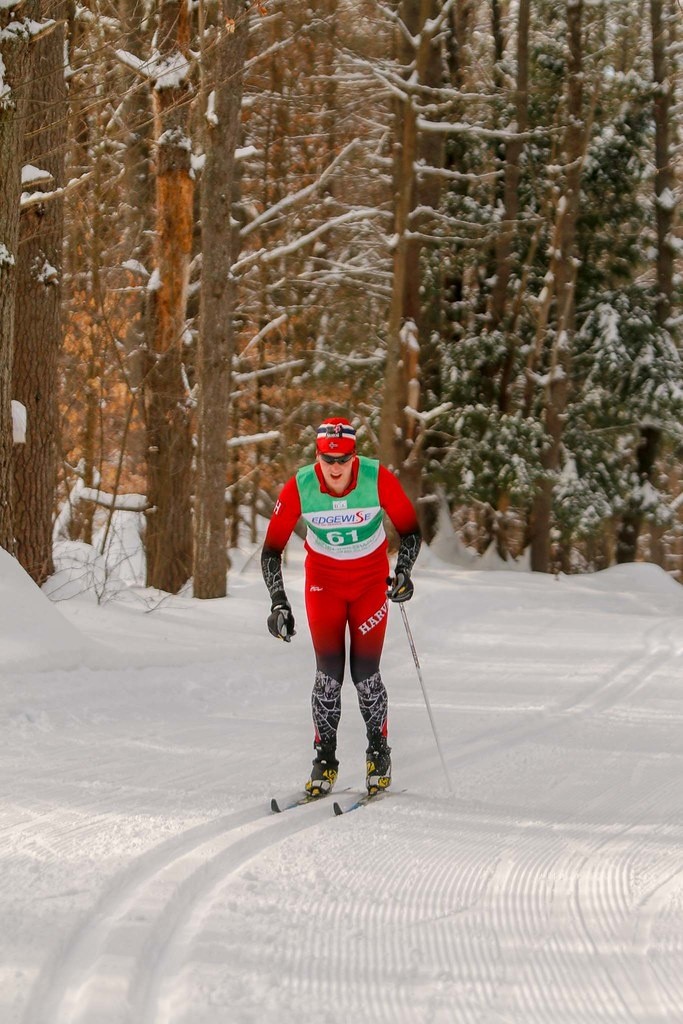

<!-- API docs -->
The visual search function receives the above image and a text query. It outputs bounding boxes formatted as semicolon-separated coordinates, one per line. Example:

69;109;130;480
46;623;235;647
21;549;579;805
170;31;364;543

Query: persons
261;417;423;795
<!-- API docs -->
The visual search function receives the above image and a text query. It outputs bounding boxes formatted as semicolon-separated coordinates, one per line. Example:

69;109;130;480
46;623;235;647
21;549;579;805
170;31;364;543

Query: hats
315;418;357;453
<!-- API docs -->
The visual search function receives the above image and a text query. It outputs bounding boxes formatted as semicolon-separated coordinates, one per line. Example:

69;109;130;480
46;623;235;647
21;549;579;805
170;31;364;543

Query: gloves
386;565;414;602
267;597;297;642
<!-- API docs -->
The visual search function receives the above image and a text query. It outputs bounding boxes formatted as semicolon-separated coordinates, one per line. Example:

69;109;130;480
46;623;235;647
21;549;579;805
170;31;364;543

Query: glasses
314;449;356;465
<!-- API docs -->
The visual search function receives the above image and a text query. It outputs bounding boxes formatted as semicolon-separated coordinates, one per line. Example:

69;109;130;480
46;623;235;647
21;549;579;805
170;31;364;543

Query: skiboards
269;788;409;817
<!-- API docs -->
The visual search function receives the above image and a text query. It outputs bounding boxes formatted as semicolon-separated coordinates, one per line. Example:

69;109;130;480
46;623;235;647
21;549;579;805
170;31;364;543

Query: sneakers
365;739;392;792
305;747;339;796
